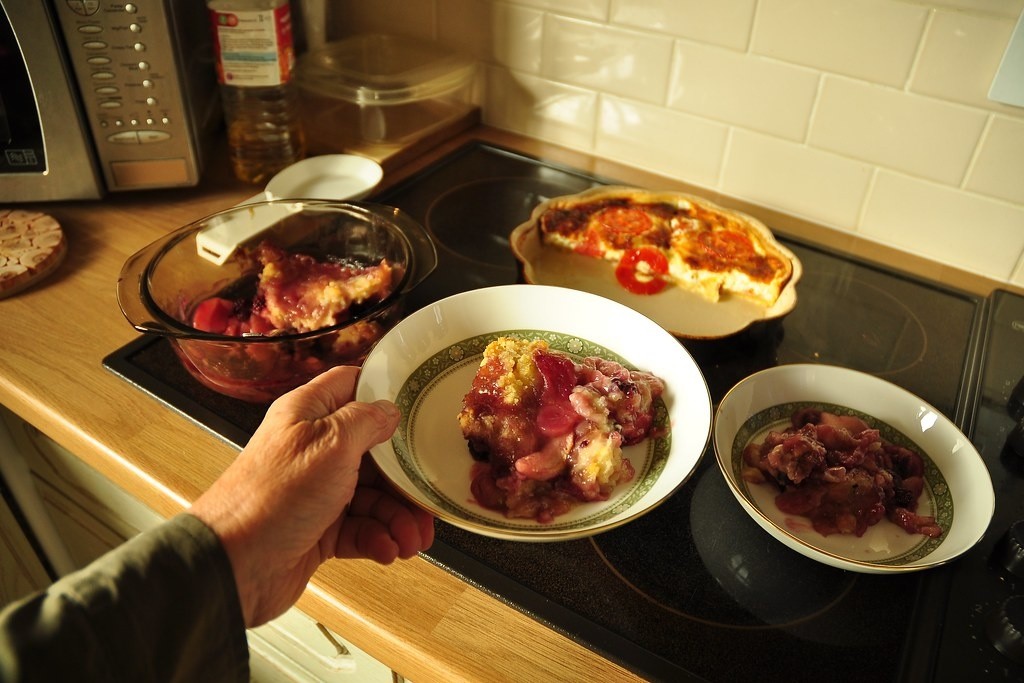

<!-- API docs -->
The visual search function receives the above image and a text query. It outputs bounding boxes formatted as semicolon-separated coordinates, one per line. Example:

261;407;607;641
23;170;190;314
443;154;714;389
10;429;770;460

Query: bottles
207;0;311;186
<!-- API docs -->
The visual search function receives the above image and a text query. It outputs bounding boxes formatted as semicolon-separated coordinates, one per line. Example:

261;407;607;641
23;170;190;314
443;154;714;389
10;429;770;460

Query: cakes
454;337;666;519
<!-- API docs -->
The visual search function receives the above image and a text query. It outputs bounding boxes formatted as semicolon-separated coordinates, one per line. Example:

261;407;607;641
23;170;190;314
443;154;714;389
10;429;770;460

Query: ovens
0;0;225;204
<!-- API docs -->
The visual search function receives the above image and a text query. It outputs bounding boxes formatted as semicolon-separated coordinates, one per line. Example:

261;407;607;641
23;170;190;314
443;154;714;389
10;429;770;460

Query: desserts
535;192;793;308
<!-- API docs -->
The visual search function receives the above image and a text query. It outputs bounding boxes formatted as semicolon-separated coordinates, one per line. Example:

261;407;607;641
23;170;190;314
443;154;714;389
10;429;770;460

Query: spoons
184;153;384;267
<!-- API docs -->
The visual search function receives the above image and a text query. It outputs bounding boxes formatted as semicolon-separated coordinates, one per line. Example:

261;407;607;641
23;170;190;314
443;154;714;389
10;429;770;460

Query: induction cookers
101;138;989;683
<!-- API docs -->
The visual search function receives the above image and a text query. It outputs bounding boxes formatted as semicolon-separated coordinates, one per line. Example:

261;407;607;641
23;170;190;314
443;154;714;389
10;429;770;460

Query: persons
0;365;435;681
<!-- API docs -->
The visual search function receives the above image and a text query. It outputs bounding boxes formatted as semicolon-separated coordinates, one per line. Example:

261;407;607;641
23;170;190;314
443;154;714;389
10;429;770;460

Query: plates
511;186;803;339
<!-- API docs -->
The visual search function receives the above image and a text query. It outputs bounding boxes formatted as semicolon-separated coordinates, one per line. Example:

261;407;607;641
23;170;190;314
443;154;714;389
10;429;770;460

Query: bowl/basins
712;364;996;573
115;196;439;402
350;283;714;541
